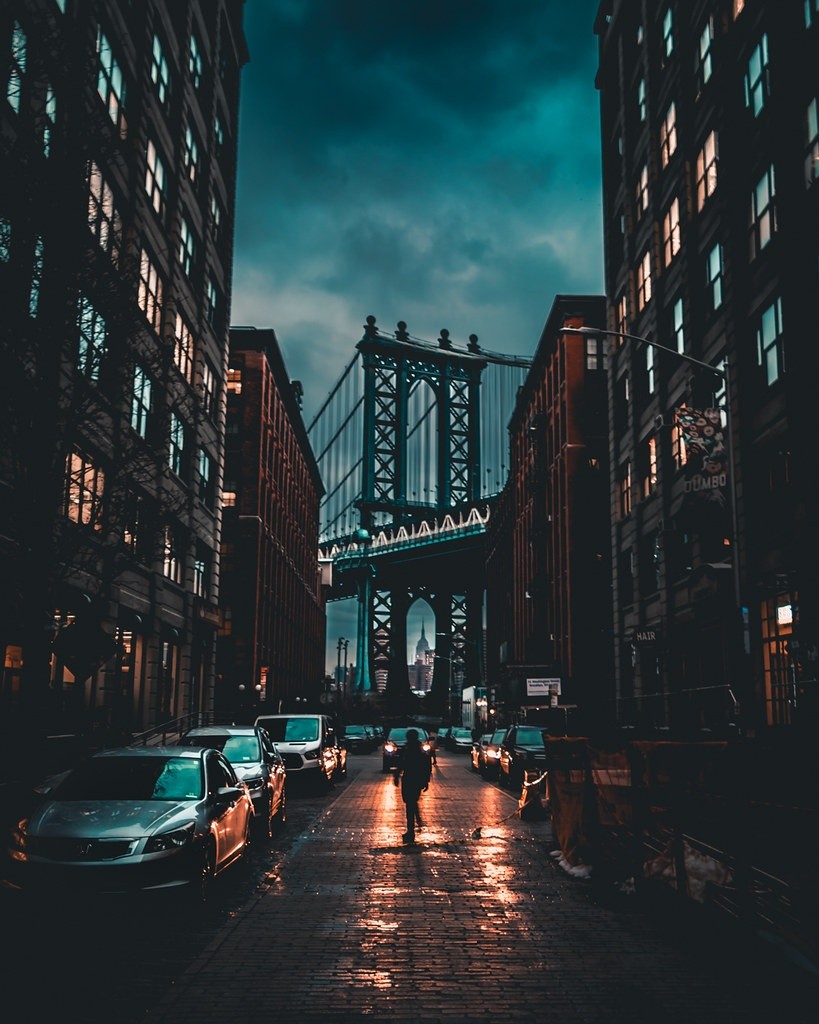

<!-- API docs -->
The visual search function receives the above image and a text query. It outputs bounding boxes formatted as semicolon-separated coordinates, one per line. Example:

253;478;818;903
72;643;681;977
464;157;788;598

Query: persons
392;729;433;843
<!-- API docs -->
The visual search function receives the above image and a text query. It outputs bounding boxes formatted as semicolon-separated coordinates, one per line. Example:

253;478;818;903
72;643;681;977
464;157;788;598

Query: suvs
251;712;347;794
496;723;548;793
482;727;508;780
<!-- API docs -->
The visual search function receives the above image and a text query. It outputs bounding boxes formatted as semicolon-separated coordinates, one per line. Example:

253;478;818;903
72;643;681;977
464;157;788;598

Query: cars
337;721;385;756
470;733;493;775
379;726;436;772
163;723;288;842
5;745;255;913
437;725;475;756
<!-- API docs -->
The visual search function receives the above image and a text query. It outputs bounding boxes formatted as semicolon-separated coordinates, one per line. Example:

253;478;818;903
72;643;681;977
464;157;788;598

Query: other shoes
402;833;414;838
417;818;422;825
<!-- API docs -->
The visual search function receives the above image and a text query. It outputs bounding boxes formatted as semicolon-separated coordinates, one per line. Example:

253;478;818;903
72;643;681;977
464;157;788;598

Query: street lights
558;325;741;625
